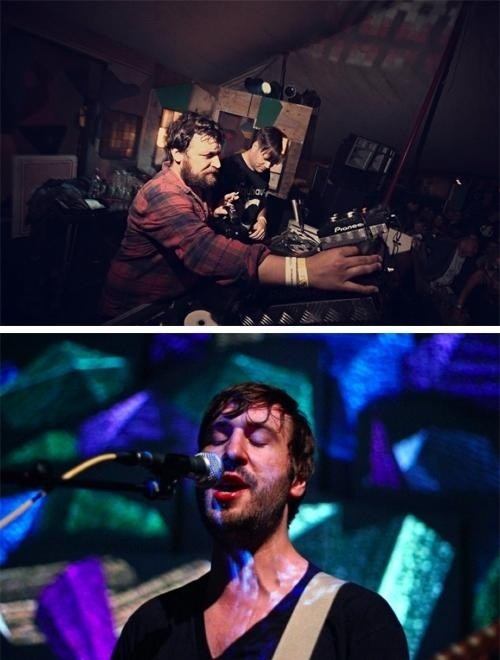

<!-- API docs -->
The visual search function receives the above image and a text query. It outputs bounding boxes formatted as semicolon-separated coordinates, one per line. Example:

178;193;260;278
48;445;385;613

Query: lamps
244;75;321;108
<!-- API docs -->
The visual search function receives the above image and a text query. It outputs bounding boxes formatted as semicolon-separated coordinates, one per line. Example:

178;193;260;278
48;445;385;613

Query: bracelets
283;255;309;289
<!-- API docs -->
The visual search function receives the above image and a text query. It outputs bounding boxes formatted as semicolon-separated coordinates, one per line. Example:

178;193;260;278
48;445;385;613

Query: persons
398;199;499;325
203;127;289;236
94;111;384;320
109;383;410;659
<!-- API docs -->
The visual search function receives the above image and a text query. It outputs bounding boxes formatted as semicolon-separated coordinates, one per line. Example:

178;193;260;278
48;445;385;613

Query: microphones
125;450;224;488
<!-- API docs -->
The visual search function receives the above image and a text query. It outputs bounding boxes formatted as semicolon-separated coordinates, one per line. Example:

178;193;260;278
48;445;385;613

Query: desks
40;186;133;265
